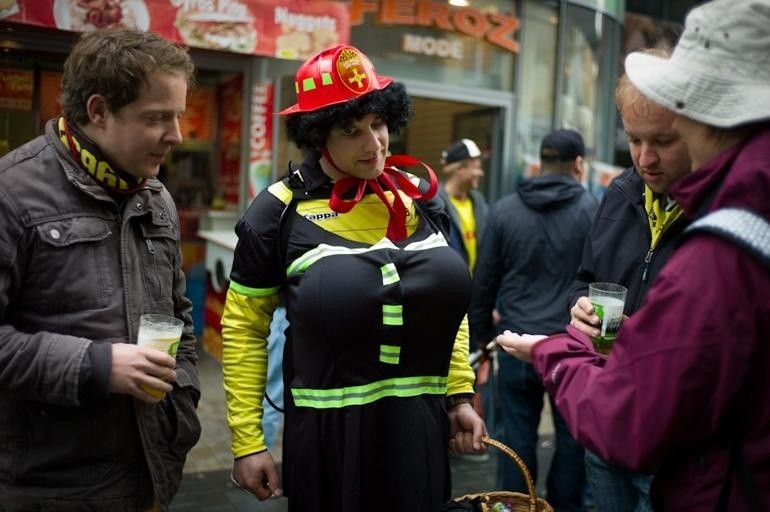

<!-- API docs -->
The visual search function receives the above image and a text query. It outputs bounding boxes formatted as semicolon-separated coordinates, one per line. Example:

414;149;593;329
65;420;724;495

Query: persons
491;1;767;512
463;124;606;510
563;42;684;512
218;40;488;510
432;134;489;421
1;21;204;512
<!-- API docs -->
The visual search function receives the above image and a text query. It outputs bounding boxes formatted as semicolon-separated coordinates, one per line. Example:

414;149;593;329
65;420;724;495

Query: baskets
450;433;551;511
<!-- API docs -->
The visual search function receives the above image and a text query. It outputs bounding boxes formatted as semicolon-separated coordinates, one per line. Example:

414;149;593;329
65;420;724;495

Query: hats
624;1;770;128
440;140;492;164
273;46;393;116
538;131;591;161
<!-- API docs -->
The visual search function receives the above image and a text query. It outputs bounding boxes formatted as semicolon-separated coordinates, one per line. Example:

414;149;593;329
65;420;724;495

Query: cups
588;282;629;352
136;315;186;403
